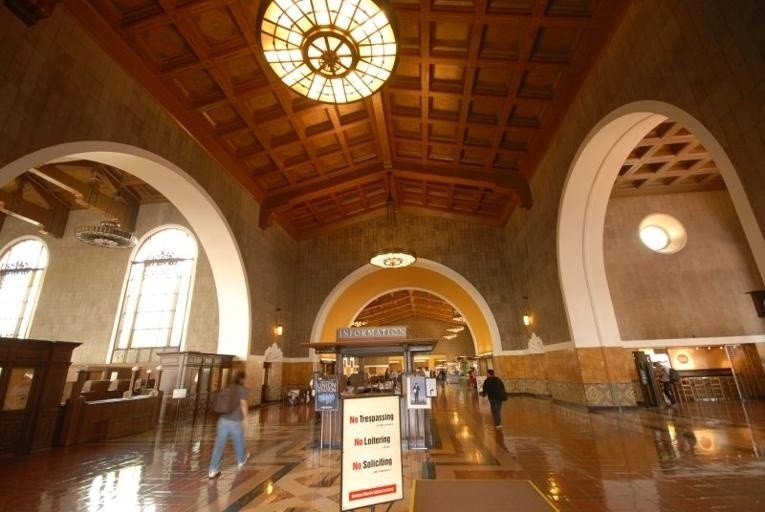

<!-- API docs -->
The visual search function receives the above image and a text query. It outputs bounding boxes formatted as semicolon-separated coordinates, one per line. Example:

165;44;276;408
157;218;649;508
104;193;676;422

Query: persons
481;370;507;428
311;371;323;425
413;382;420;404
208;371;248;481
652;362;676;404
437;367;447;397
390;369;398;384
493;430;517;478
208;471;246;511
684;431;697;452
468;367;476;388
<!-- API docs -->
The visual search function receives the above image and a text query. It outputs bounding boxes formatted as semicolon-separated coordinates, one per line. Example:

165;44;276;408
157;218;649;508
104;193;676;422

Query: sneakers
208;468;221;478
238;452;250;467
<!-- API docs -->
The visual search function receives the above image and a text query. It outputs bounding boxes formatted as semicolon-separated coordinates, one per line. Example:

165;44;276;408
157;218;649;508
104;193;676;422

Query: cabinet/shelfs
156;350;236;427
0;337;84;458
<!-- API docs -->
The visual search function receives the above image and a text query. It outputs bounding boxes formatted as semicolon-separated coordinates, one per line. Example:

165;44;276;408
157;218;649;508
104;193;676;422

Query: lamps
258;0;399;106
275;307;283;337
520;295;531;328
367;171;417;271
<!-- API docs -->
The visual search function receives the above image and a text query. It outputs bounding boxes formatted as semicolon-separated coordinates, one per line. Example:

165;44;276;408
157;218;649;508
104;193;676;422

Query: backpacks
669;368;679;382
212;387;237;413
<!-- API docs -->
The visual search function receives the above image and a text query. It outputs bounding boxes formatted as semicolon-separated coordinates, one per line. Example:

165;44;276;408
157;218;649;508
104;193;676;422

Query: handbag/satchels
500;392;507;401
